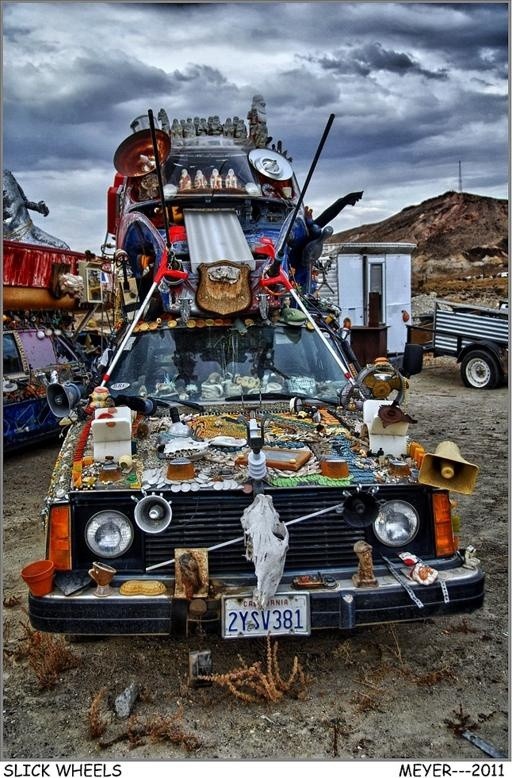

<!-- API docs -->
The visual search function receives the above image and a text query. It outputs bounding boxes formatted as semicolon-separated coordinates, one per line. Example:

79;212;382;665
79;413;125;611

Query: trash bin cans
402;344;424;374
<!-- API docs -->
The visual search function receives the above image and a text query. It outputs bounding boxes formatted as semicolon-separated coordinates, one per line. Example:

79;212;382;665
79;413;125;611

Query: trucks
432;300;508;388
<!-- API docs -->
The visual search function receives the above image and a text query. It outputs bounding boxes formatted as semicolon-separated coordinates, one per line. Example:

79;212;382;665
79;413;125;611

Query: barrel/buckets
20;560;55;597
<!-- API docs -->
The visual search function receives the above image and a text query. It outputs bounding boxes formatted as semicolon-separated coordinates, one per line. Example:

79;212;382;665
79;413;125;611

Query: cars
29;315;485;643
4;327;87;450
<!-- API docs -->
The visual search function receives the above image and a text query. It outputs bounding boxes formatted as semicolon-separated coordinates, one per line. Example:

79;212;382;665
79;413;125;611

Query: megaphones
46;383;81;418
418;441;479;497
129;489;173;535
342;491;380;529
